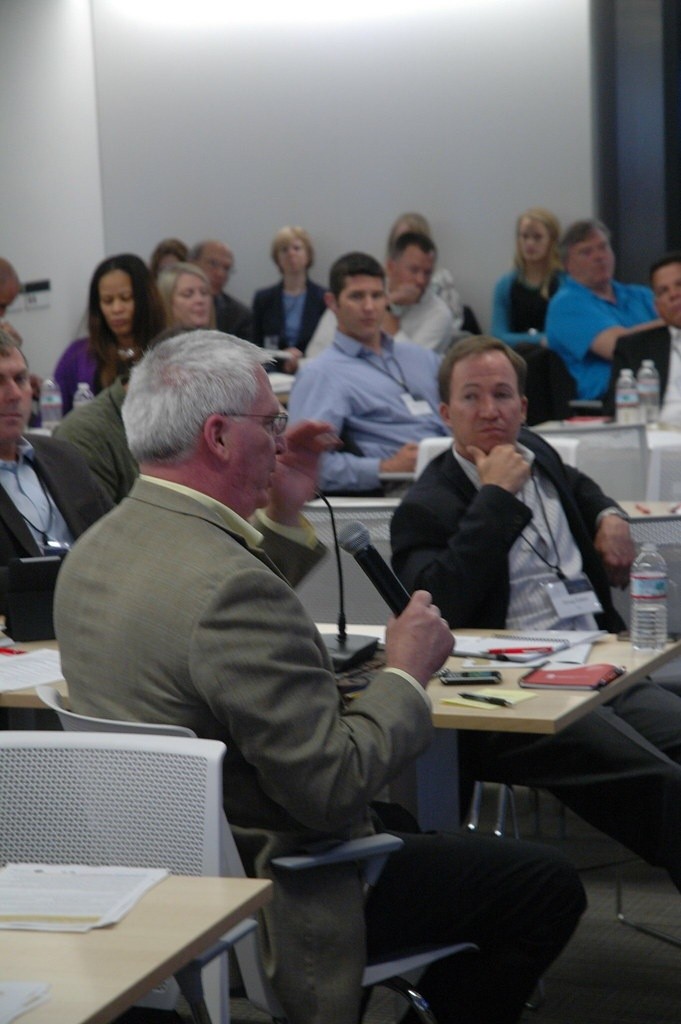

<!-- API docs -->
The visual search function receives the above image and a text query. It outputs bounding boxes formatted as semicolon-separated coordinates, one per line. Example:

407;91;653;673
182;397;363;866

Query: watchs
596;507;627;526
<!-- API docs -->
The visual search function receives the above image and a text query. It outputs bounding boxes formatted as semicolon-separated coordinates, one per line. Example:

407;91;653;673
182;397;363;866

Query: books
450;627;607;663
518;663;618;691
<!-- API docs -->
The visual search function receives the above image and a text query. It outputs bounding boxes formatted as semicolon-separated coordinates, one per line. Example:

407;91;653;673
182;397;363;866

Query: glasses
221;412;288;436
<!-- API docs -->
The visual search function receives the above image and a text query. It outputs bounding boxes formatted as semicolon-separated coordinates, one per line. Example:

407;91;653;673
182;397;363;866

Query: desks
414;416;679;504
0;627;681;840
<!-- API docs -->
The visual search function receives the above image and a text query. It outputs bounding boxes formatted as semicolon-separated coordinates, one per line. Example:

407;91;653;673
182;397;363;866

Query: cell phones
440;670;502;685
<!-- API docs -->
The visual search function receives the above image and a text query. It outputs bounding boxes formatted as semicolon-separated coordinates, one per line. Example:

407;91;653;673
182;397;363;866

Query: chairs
0;686;482;1024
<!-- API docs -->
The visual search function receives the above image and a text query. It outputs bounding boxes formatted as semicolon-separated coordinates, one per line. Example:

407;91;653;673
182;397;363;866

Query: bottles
615;359;660;428
630;542;668;653
72;381;94;409
38;374;63;431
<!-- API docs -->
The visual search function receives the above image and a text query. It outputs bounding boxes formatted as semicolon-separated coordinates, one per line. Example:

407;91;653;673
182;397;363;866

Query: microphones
336;521;411;617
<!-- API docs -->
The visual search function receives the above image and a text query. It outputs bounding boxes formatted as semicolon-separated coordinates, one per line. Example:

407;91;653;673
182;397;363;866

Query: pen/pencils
457;691;512;708
670;505;681;513
636;504;650;514
481;647;555;655
0;647;27;655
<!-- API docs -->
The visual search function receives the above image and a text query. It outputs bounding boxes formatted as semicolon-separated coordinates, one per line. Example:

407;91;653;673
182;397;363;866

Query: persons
1;206;681;569
53;332;587;1024
390;338;680;886
